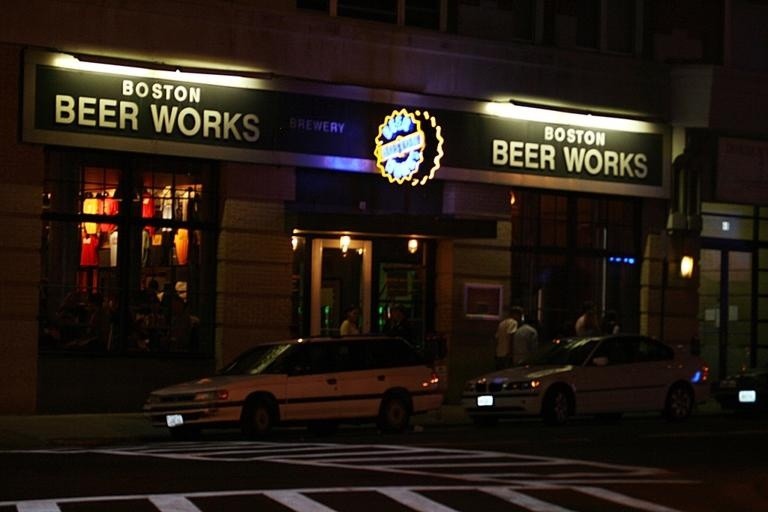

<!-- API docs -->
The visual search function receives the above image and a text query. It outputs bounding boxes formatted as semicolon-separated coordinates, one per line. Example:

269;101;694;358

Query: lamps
291;238;298;252
680;254;694;280
407;239;418;254
339;236;351;257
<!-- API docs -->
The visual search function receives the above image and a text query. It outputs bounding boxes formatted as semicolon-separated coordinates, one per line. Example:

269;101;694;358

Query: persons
52;275;206;383
494;301;620;369
320;300;413;345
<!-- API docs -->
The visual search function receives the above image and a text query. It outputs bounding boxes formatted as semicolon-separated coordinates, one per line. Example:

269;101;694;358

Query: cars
715;368;768;416
458;330;714;428
137;329;446;442
